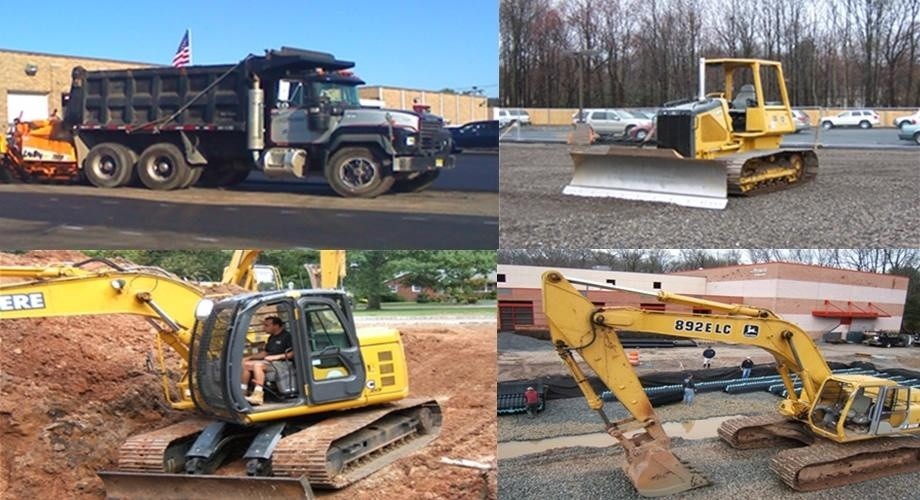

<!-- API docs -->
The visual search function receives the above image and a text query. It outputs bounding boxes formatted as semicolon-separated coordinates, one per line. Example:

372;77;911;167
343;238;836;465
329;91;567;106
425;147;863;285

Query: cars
793;107;810;133
823;109;919;143
575;108;659;141
862;336;891;349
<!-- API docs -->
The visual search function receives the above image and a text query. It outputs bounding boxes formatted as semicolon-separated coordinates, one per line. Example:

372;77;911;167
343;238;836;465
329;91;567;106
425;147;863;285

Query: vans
498;107;529;124
448;120;497;153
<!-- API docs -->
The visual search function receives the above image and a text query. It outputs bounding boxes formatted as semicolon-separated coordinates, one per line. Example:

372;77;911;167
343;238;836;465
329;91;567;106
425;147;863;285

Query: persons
524;386;538;419
740;356;754;378
702;345;715;369
681;372;697;407
240;316;295;406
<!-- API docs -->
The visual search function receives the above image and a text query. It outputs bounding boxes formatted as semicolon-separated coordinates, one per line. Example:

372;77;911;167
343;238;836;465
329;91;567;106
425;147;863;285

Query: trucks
71;45;455;200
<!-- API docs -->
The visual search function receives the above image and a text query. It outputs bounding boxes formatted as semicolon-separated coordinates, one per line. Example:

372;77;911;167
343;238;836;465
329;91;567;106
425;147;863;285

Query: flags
171;29;190;68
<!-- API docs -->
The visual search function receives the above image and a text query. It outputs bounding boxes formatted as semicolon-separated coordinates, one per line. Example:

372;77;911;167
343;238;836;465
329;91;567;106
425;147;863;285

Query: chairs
843;396;871;424
728;84;755;113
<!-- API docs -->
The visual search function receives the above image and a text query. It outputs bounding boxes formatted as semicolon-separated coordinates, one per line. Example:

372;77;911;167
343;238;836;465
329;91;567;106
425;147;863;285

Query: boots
244;389;264;405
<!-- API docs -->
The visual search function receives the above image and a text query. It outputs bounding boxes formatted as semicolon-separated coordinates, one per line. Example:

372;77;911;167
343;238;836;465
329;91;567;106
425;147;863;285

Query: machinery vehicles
6;118;76;181
562;56;820;210
0;246;443;500
541;270;919;498
